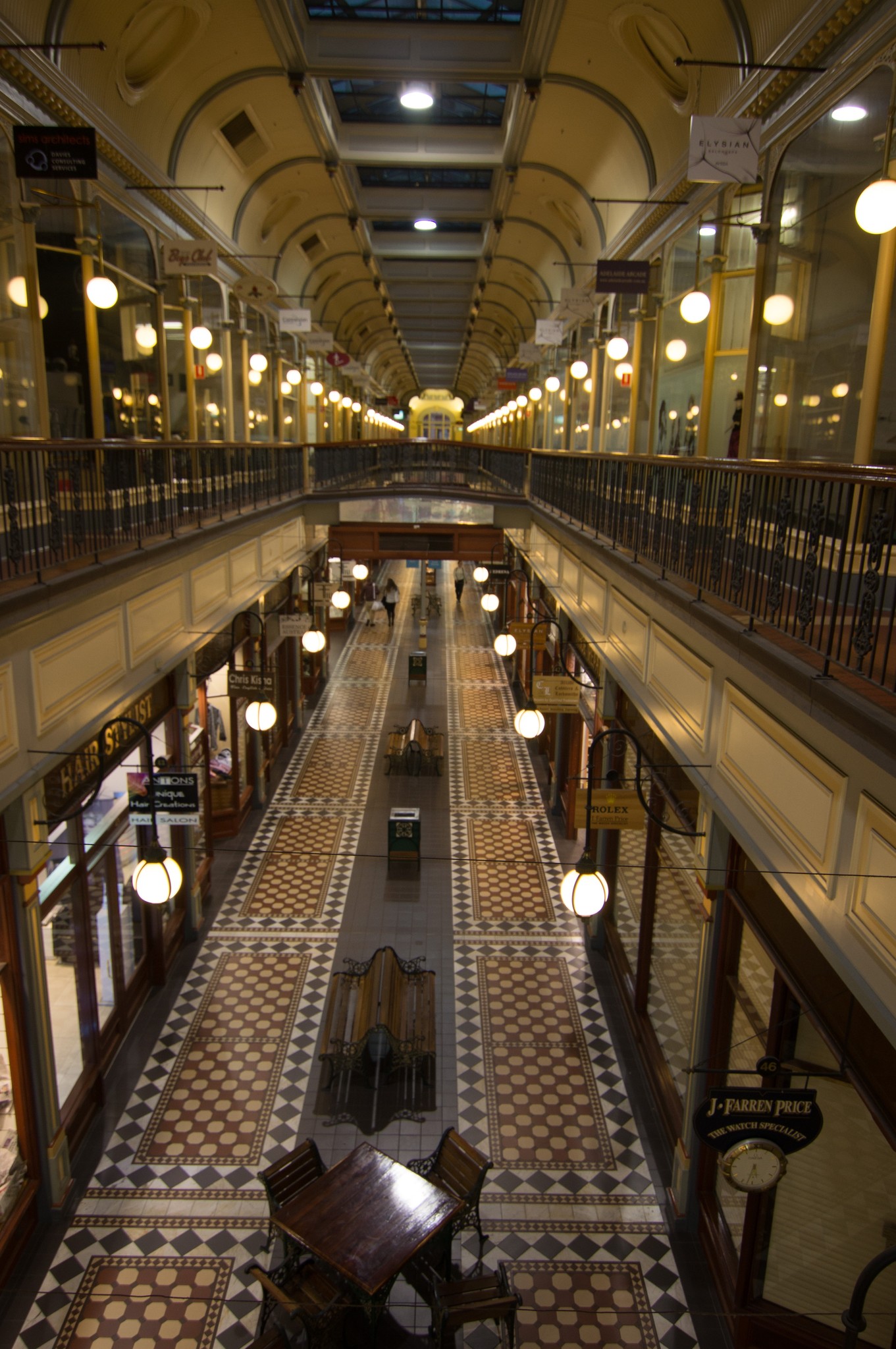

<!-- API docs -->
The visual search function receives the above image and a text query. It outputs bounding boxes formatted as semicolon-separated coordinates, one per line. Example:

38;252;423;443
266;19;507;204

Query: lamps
354;562;370;580
471;560;490;584
330;584;353;610
559;857;611;920
513;700;548;738
481;589;499;611
301;622;327;652
494;625;518;657
243;692;279;732
131;836;184;904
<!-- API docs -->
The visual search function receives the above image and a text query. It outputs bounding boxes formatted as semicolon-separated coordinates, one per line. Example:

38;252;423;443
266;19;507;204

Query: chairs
249;1254;362;1348
405;1126;494;1252
258;1137;331;1274
428;1260;525;1347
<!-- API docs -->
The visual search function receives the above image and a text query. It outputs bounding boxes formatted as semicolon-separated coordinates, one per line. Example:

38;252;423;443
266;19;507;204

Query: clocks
718;1139;789;1196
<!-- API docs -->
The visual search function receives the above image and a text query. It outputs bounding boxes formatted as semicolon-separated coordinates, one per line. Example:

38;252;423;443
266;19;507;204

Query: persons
682;394;697;456
454;560;468;604
724;390;745;459
655;399;668;455
668;406;681;456
359;573;382;627
380;579;401;627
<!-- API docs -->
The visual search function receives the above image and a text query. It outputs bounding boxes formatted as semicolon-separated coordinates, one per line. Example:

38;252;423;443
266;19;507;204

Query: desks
271;1139;464;1319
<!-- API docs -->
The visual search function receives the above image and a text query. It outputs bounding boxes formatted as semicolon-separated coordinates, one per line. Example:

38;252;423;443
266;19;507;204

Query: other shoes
389;623;394;626
366;619;370;626
457;598;461;602
370;623;376;626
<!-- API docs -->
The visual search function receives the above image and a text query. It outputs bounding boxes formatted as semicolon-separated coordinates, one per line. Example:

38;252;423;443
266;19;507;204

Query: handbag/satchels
395;591;399;603
372;600;383;611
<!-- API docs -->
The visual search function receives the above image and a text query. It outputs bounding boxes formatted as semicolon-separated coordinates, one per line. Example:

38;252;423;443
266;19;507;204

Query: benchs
425;590;443;617
414;720;445;777
316;943;384;1094
384;719;415;776
377;946;439;1088
412;591;421;615
